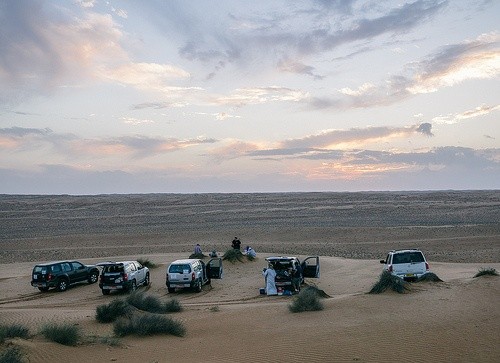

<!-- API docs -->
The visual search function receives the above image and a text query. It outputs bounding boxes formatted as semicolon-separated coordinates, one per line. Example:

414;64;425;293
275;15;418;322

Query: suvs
31;259;100;290
166;256;223;291
264;255;320;292
379;248;430;281
96;260;150;294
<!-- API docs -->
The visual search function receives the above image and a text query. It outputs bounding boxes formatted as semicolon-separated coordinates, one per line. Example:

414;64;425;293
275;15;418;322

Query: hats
196;244;200;246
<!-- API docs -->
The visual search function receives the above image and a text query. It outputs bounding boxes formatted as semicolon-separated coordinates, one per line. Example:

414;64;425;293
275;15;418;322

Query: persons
209;250;218;259
231;236;241;250
262;263;278;296
194;243;203;255
245;245;256;257
291;262;302;293
